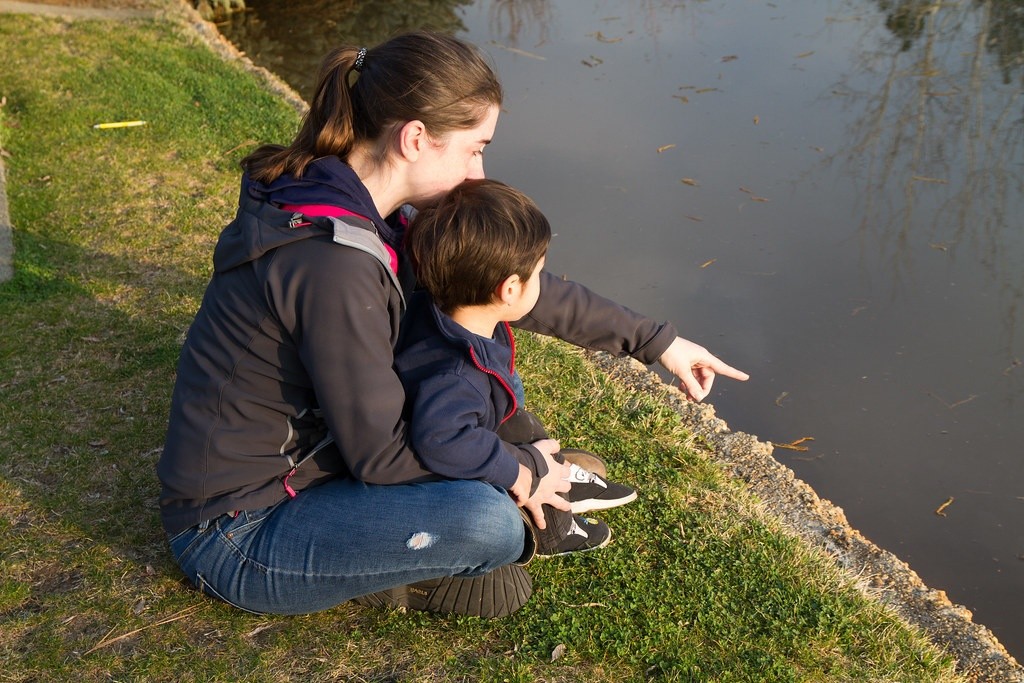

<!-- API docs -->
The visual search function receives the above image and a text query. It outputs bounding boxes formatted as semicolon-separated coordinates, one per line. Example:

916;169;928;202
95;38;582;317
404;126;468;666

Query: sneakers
567;472;637;513
537;514;613;558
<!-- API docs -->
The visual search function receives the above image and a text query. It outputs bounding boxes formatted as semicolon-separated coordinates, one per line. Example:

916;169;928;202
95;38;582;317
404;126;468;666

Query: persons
156;33;752;619
391;179;639;561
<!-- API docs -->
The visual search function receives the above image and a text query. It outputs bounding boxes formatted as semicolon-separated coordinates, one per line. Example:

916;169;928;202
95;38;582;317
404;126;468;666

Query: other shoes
356;564;533;619
